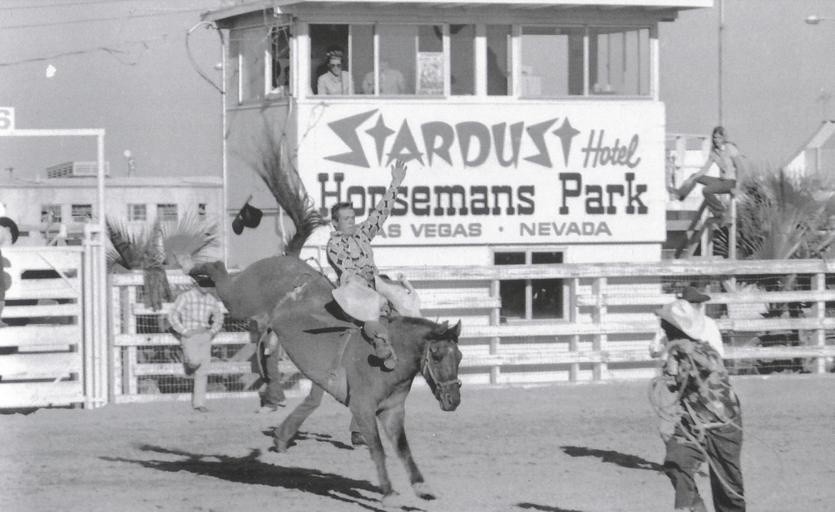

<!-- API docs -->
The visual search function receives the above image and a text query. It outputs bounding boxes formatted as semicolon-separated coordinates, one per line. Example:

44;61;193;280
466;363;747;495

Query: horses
172;117;463;508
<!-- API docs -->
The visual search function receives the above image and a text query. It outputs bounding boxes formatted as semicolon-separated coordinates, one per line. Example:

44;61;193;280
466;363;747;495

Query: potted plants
711;169;834;374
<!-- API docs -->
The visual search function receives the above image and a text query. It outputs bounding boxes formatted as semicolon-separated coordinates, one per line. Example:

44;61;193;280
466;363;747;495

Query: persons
654;299;746;512
317;49;355;96
362;52;406;95
326;159;420;361
168;273;225;413
648;286;723;445
667;127;743;230
258;331;288;414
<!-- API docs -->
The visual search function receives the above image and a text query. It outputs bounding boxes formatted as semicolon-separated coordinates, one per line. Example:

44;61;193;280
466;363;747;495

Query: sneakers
364;320;391;360
197;406;208;411
256;400;286;414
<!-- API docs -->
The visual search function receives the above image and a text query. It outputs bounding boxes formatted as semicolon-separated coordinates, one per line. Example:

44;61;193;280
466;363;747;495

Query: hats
651;299;703;341
675;286;711;302
233;194;263;235
189;272;215;287
